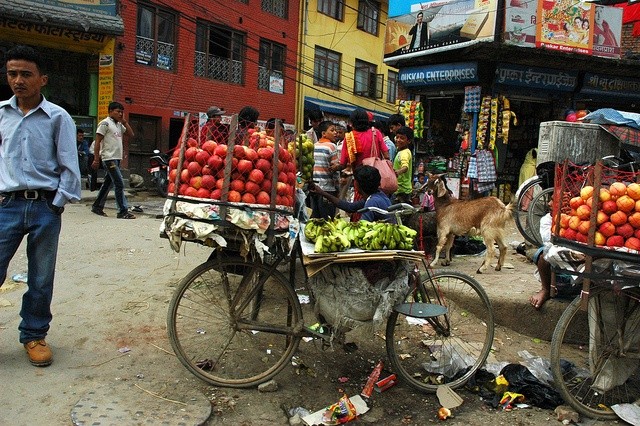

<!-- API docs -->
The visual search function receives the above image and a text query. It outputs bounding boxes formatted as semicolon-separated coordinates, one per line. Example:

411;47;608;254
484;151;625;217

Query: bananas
363;219;418;251
327;215;335;227
334;213;348;230
343;220;369;247
314;228;349;252
304;218;336;242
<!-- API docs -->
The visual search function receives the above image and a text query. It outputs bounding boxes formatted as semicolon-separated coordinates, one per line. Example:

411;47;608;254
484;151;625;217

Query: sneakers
117;212;136;219
91;209;107;217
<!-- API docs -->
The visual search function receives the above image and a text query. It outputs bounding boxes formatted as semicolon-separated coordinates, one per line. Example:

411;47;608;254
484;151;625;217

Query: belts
3;190;56;200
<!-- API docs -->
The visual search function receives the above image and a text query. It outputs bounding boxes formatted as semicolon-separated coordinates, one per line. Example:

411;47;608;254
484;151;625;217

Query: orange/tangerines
288;134;315;180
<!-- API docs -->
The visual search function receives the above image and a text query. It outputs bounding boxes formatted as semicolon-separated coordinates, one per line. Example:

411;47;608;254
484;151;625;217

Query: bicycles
528;160;640;248
512;155;624;247
245;219;275;320
549;258;640;420
166;201;494;394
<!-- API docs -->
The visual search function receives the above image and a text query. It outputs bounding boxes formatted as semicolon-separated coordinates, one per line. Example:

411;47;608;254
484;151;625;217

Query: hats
207;106;226;116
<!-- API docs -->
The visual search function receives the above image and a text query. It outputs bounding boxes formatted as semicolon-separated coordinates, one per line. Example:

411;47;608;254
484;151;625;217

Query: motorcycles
149;149;170;197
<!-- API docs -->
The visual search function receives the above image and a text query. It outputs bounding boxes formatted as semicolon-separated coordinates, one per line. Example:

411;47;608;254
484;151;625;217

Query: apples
168;129;296;206
566;110;587;123
437;408;450;419
552;182;640;251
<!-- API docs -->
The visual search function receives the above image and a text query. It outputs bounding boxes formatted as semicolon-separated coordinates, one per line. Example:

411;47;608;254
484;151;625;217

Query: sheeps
419;170;520;275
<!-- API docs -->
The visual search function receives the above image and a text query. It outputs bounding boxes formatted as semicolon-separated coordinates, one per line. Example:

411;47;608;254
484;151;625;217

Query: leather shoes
24;338;53;366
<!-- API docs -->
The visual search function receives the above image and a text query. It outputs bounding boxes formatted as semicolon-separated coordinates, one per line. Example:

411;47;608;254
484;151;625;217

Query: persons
392;126;414;205
265;117;284;136
414;173;425;189
91;102;136;219
235;106;260;146
200;106;228;145
304;110;324;144
76;128;90;179
88;140;102;159
563;23;574;41
409;12;428;49
313;121;340;220
335;121;348;164
517;146;537;212
1;45;83;368
574;17;586;45
373;121;397;163
413;163;428;185
582;19;589;43
529;191;571;308
339;108;398;222
310;164;396;224
387;114;405;136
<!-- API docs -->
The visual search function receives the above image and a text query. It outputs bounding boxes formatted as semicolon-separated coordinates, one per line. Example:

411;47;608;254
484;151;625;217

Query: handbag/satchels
362;154;398;194
